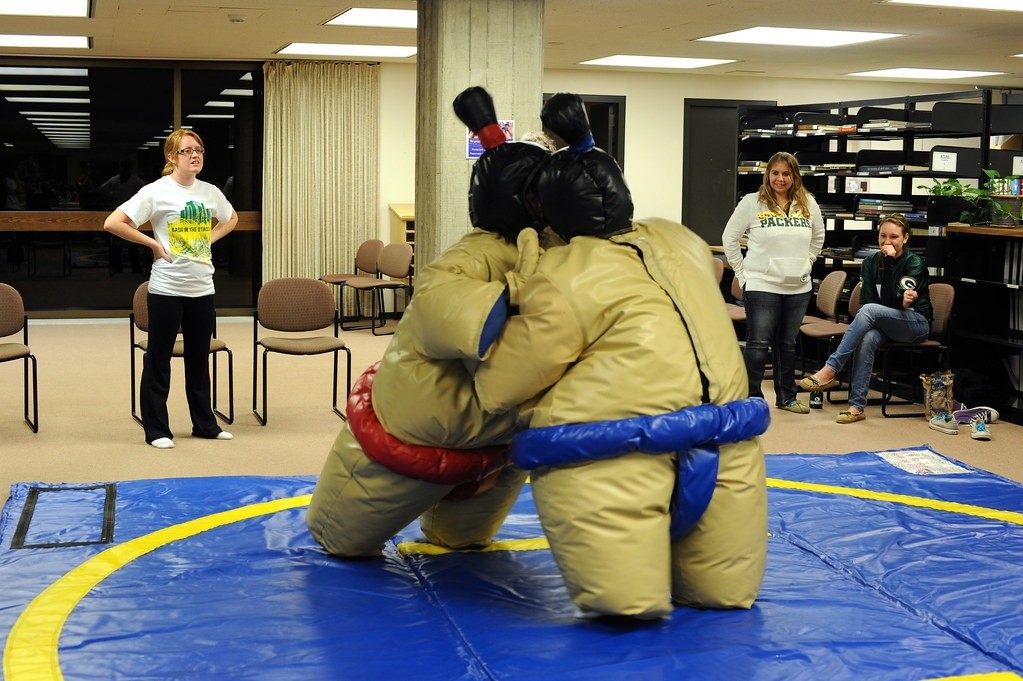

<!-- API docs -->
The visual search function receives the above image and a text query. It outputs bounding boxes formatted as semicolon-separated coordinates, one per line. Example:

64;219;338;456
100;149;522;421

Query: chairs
0;283;38;434
319;240;385;330
27;232;72;279
252;278;351;425
715;258;956;416
130;282;234;430
340;243;414;337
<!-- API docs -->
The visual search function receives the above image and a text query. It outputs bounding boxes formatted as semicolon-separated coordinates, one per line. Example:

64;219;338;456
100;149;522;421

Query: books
821;244;881;259
742;119;931;135
819;199;927;222
799;163;929;173
738;161;768;174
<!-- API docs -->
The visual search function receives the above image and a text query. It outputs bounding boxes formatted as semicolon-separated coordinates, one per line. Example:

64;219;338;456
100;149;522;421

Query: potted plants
960;168;1023;226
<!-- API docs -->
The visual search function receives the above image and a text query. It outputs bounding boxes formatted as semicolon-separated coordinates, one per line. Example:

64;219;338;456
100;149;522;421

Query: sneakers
953;406;999;424
930;413;959;435
971;420;991;439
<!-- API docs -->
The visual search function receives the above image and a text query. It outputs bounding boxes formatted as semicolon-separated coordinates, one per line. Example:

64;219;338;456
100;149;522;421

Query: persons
721;152;825;413
99;157;145;274
74;159;98;211
104;130;238;449
799;213;934;424
3;157;26;264
43;179;79;202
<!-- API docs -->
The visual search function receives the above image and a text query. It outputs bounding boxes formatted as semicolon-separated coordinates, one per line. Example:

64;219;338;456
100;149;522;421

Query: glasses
176;147;205;156
879;214;909;232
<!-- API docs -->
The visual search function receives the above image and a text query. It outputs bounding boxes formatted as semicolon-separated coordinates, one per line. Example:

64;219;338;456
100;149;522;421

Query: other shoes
779;400;810;414
836;410;865;423
800;375;836;391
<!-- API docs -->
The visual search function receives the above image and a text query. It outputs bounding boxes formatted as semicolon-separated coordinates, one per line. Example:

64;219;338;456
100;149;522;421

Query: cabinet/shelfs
387;200;415;303
737;84;1023;423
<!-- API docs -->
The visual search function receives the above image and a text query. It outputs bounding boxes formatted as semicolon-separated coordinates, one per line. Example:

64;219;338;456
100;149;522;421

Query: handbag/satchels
920;371;954;421
